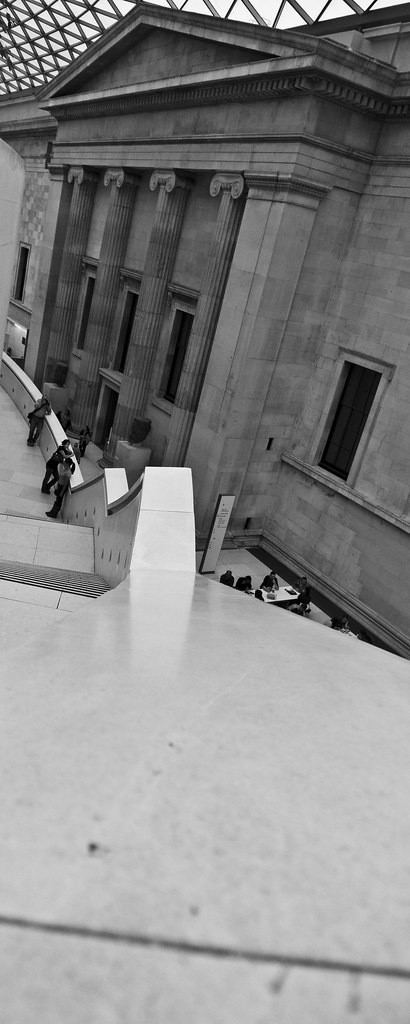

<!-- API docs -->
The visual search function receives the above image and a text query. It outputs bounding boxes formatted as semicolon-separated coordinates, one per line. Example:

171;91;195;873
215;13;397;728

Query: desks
242;586;301;608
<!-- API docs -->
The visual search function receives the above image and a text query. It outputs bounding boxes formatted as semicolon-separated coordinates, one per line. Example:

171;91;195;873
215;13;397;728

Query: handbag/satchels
27;412;33;419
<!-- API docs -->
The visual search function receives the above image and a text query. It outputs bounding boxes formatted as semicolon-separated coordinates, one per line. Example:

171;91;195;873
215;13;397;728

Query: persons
219;570;234;587
355;630;371;644
255;590;264;601
56;409;71;433
72;443;80;465
41;440;75;495
297;587;311;615
236;576;252;591
26;394;52;447
79;425;91;457
331;615;349;632
292;577;310;593
260;571;279;592
46;458;75;518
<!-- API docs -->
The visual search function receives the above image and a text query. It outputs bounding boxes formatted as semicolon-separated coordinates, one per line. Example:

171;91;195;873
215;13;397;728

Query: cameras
44;400;51;407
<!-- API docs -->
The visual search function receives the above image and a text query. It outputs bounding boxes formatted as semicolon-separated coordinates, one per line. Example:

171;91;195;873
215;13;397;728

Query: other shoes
46;512;56;518
42;488;50;494
27;440;34;446
